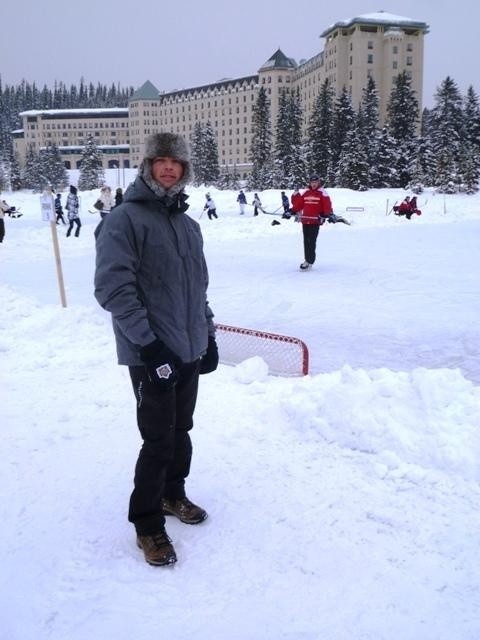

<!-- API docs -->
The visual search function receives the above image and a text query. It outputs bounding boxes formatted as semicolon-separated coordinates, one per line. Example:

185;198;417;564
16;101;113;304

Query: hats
70;185;77;195
138;133;193;196
309;174;320;187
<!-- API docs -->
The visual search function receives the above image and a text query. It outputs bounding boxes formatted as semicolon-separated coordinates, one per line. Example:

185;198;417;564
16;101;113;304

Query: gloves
327;214;336;223
139;338;183;393
199;336;218;374
282;209;292;219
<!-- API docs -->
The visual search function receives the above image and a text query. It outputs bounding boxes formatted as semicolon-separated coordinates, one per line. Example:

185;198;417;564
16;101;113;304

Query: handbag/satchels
94;201;103;210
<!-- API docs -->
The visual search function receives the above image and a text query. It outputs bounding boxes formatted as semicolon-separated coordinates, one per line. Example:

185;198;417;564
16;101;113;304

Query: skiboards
88;210;109;214
258;204;317;220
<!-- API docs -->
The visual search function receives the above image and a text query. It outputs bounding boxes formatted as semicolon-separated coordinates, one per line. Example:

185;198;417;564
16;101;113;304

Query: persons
282;173;337;271
409;196;418;213
54;193;67;225
93;187;125;221
65;185;82;237
204;192;218;220
0;189;8;243
291;184;303;215
252;193;262;216
281;192;289;211
399;196;412;219
237;190;247;215
90;132;219;566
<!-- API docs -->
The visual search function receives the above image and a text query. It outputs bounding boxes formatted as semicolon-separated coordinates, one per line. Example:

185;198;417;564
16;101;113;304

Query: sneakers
136;526;177;566
160;495;208;523
300;261;310;269
